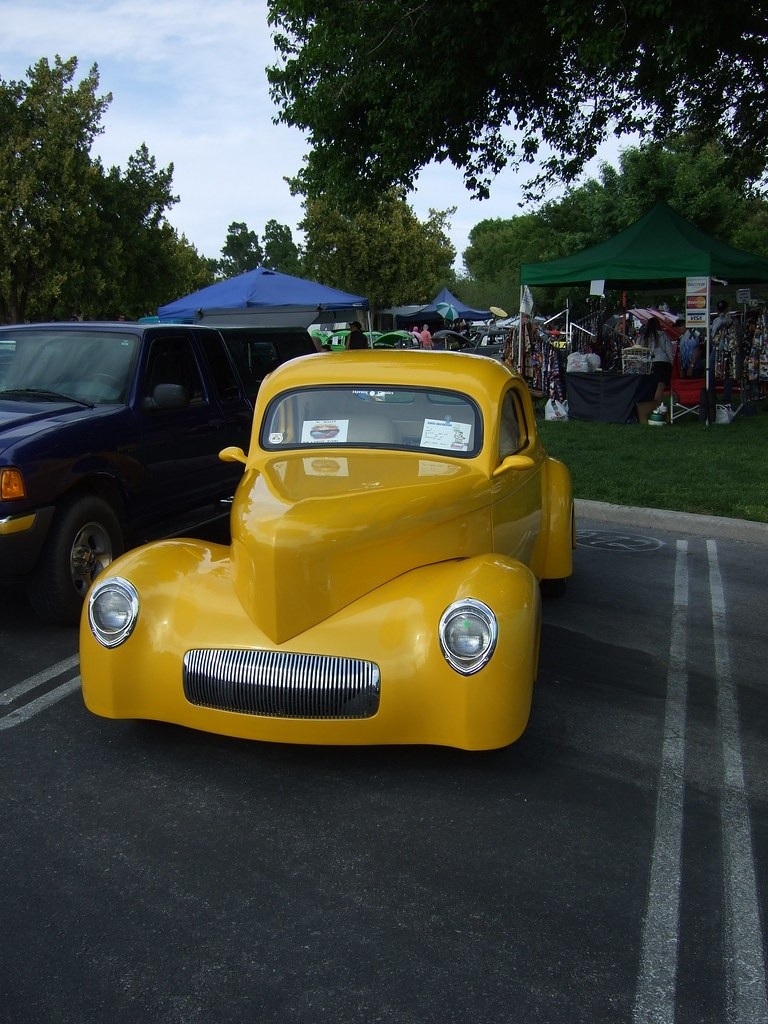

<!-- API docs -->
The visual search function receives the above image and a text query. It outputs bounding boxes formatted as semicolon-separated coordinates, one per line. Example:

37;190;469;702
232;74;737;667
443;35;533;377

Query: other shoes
653;402;668;416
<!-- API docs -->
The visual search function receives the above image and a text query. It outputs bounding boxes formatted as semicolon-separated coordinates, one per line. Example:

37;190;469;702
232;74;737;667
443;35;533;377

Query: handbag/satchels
567;352;601;372
714;404;733;424
544;398;569;422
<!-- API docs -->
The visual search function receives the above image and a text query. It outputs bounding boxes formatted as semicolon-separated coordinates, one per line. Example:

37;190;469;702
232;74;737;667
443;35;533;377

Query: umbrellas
490;307;508;317
436;302;459;320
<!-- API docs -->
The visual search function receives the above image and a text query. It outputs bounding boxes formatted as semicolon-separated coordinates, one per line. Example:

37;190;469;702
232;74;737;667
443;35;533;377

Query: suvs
0;320;319;632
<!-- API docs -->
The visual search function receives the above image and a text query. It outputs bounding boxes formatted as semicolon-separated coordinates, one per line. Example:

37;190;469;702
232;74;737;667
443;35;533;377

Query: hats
673;319;685;327
717;300;727;311
349;321;361;329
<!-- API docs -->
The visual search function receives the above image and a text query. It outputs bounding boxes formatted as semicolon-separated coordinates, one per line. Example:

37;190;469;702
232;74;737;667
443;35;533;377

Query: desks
563;371;660;426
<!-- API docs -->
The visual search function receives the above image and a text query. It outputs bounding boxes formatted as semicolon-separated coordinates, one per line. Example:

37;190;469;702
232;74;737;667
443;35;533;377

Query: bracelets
690;364;694;367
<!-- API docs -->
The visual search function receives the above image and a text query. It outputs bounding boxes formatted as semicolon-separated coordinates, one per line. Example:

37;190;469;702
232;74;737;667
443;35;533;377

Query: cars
79;348;578;752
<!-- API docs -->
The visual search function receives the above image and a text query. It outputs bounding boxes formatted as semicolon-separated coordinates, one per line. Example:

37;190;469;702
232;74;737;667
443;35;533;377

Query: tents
518;196;768;427
157;266;374;349
378;287;552;332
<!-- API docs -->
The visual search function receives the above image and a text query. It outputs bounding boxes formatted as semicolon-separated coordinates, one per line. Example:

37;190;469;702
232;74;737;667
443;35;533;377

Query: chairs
529;388;551;419
670;377;710;428
318;412;403;445
443;412;517;456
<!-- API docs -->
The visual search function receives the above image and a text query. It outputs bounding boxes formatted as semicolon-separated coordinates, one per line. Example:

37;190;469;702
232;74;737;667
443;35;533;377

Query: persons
411;323;434;349
312;336;334;352
709;300;733;403
117;314;126;321
673;317;707;378
458;325;470;347
346;320;368;349
636;319;675;403
